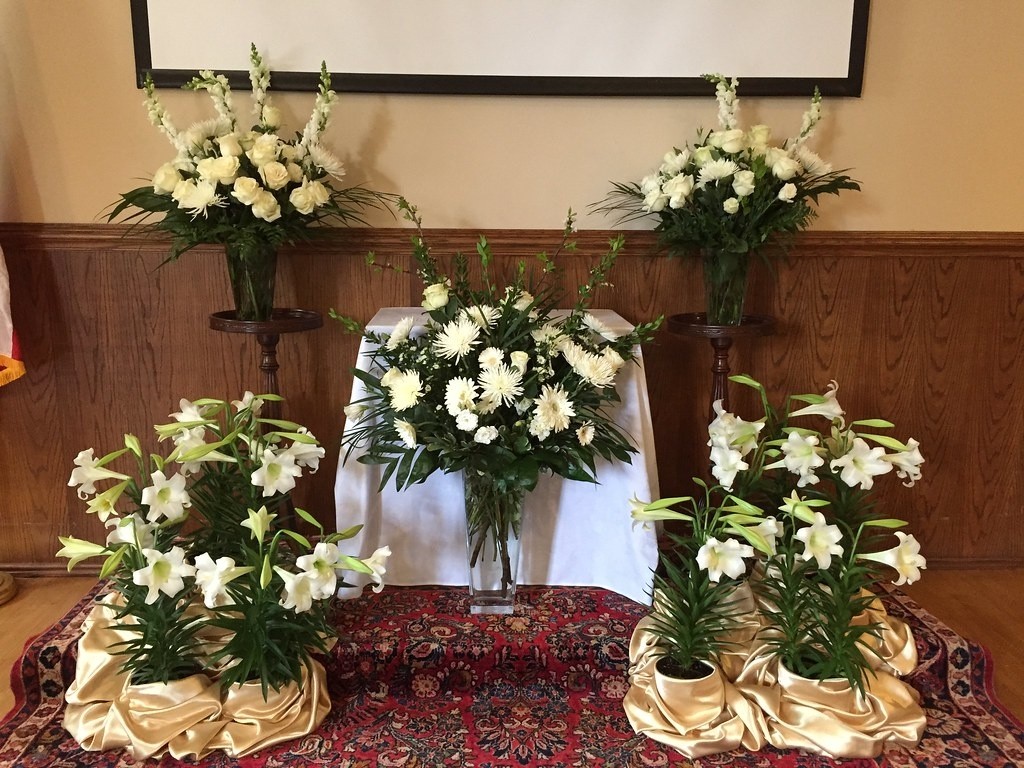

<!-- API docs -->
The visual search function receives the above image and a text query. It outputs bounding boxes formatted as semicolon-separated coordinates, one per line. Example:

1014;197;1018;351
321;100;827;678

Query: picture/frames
131;0;869;98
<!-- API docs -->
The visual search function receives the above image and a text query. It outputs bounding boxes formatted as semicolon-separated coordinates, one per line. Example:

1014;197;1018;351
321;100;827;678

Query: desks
334;311;667;605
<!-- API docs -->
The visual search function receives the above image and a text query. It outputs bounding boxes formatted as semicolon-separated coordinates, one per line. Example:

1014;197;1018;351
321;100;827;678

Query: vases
222;242;279;318
699;245;752;326
646;653;727;729
774;654;859;706
460;474;523;615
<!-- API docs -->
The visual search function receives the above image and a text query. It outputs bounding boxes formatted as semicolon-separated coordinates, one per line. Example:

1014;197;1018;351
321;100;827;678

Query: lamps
0;243;27;605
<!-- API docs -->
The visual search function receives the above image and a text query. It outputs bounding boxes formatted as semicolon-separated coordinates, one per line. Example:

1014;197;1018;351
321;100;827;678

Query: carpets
0;564;1024;768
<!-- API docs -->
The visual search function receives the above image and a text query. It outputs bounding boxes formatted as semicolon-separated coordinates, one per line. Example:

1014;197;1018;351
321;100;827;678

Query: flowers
90;39;400;280
52;388;396;682
586;70;864;265
326;193;664;497
626;377;928;664
